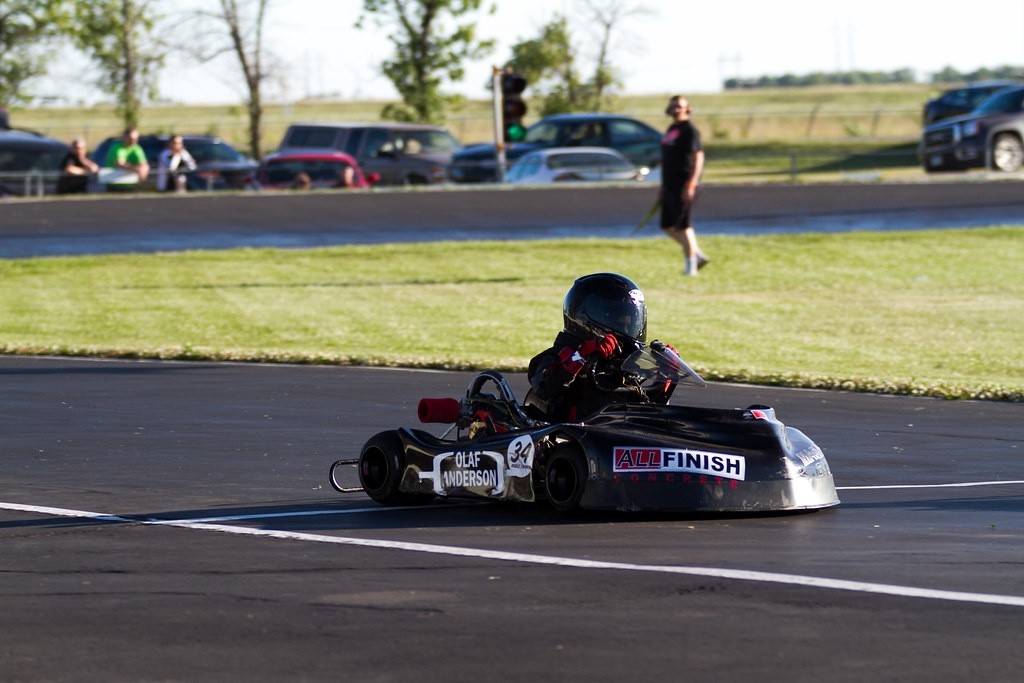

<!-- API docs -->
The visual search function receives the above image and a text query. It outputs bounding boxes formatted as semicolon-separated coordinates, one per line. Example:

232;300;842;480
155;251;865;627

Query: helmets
563;273;648;352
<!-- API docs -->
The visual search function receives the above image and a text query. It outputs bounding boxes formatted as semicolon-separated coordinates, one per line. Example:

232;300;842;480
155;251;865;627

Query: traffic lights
502;73;529;141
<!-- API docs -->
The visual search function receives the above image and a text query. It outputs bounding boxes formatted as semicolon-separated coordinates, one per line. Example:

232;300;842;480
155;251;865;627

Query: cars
501;147;649;183
921;78;1024;173
447;115;666;183
242;148;380;192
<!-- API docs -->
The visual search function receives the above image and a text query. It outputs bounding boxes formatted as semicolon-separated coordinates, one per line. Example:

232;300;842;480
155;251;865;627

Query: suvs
0;128;73;194
88;134;258;191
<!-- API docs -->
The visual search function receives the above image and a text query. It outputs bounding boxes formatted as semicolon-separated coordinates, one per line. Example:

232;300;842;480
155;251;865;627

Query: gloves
650;339;681;378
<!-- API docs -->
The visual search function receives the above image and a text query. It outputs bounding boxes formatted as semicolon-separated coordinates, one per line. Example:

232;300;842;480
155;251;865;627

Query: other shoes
680;254;710;277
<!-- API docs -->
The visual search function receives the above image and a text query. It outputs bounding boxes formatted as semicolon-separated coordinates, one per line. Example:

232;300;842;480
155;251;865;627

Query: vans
278;124;464;184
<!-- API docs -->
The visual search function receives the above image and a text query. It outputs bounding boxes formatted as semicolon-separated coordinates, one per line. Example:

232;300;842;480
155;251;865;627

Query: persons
297;173;310;188
528;273;680;424
658;96;708;276
156;135;197;192
332;166;354;188
57;140;99;195
105;128;150;193
